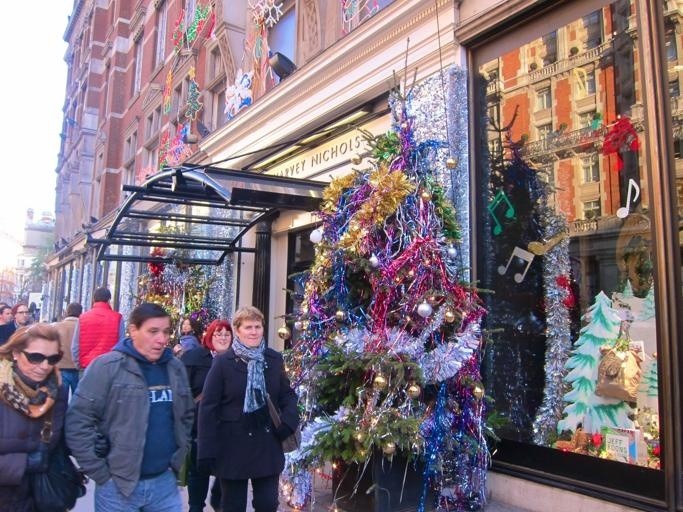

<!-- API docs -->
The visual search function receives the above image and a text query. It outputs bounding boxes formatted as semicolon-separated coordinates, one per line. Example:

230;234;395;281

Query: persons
0;324;83;510
63;302;195;512
0;302;34;348
194;304;303;512
171;318;200;359
70;286;127;383
0;303;9;310
0;305;13;327
182;319;235;512
56;302;83;415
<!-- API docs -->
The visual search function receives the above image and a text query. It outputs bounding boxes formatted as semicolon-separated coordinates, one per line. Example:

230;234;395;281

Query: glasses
212;332;230;338
19;350;62;365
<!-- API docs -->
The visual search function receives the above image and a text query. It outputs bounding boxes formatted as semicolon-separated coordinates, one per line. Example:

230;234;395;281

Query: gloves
24;451;48;474
276;422;291;442
94;433;109;458
196;459;211;478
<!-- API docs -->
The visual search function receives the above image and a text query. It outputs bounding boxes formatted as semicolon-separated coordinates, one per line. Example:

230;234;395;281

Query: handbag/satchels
266;412;301;452
32;442;86;512
